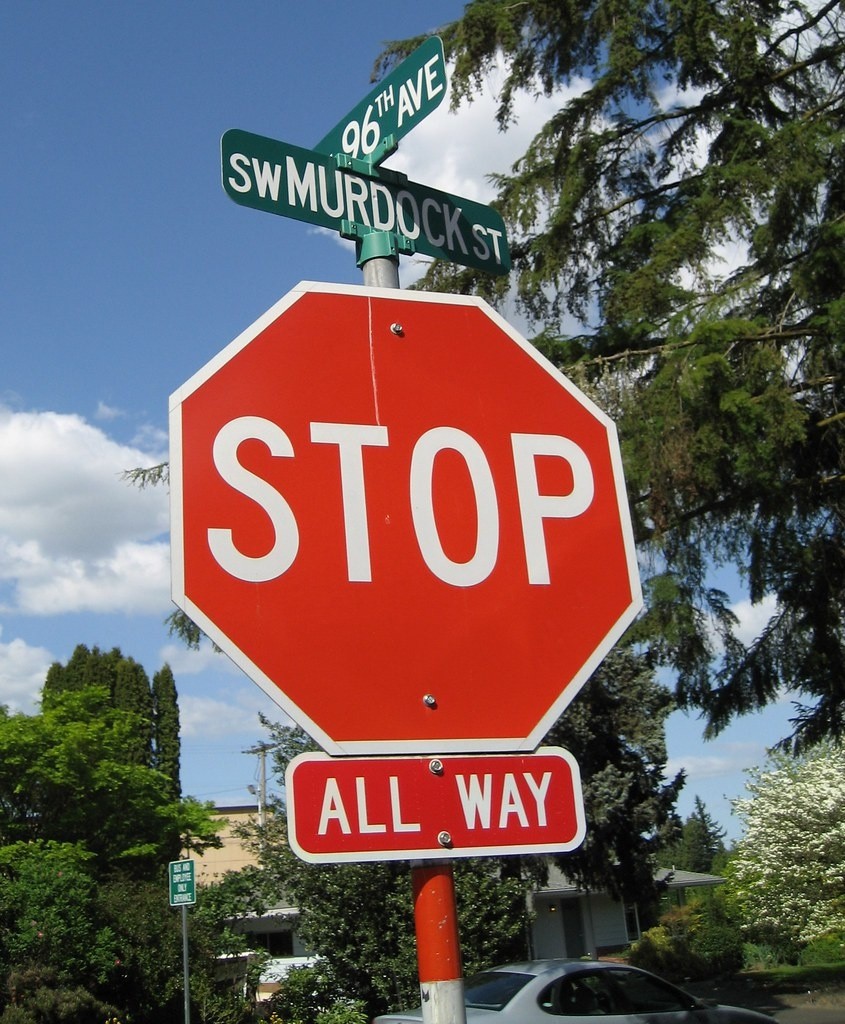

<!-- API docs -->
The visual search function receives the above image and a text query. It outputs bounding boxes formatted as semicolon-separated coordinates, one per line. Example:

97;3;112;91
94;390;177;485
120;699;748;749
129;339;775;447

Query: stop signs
167;280;644;757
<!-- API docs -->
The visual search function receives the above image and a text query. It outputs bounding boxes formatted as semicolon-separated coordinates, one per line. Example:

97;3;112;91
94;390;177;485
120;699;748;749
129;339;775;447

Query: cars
374;959;777;1023
